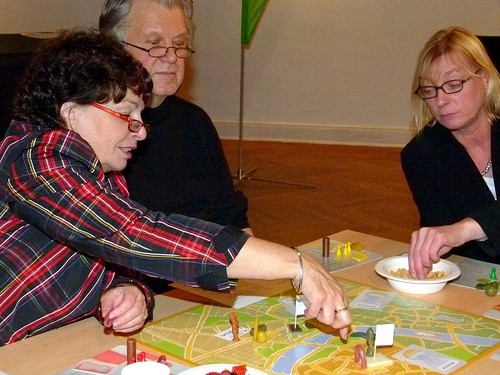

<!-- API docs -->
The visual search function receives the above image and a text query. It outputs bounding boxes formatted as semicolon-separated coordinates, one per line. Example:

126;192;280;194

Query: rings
336;307;349;313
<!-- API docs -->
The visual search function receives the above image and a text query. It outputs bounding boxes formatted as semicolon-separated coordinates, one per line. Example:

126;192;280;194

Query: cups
121;361;170;375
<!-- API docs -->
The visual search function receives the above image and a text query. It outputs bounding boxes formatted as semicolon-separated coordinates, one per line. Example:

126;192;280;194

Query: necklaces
481;159;492;175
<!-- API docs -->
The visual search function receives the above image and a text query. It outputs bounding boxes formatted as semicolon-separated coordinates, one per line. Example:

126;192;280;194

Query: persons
400;26;500;280
99;0;255;239
0;27;352;346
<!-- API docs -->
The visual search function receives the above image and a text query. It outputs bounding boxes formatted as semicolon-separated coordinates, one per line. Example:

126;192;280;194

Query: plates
176;363;268;375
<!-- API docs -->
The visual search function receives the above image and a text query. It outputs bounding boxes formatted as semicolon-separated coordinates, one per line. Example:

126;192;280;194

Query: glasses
415;68;481;99
122;41;195;58
83;101;151;138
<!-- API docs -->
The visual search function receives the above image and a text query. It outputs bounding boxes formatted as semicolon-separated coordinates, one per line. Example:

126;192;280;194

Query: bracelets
288;246;304;293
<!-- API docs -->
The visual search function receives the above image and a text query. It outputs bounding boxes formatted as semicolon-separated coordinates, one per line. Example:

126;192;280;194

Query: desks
0;229;500;375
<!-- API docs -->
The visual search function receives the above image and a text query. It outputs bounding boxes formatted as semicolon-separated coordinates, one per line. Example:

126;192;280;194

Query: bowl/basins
374;255;461;295
